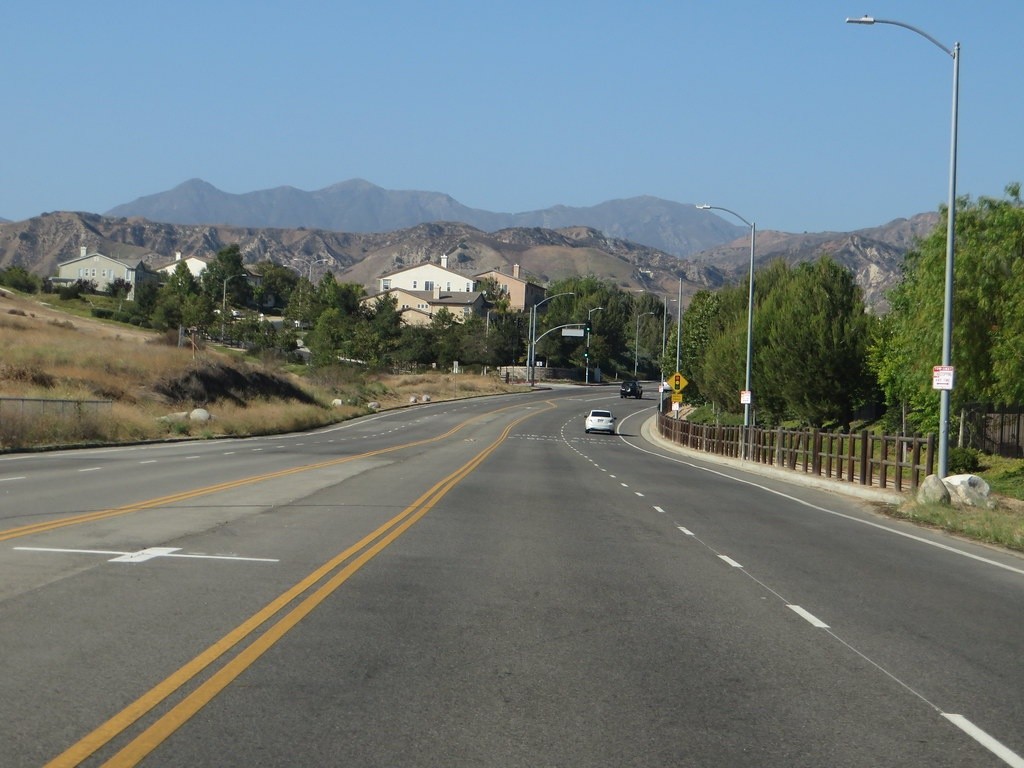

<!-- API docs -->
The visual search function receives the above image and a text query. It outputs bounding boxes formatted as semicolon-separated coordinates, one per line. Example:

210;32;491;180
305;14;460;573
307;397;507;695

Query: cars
663;378;673;391
583;410;617;434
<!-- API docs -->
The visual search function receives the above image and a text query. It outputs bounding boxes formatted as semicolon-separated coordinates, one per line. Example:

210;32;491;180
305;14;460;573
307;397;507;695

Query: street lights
844;13;961;480
295;258;329;304
635;289;668;413
531;292;574;386
282;263;312;313
640;268;683;420
585;307;604;384
695;204;756;460
221;273;247;345
634;312;654;376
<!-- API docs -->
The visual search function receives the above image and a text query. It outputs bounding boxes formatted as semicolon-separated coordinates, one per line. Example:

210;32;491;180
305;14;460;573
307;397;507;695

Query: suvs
620;380;642;399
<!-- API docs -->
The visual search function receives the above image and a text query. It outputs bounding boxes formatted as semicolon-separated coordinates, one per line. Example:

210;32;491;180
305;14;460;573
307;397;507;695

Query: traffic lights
584;320;592;332
584;346;589;358
